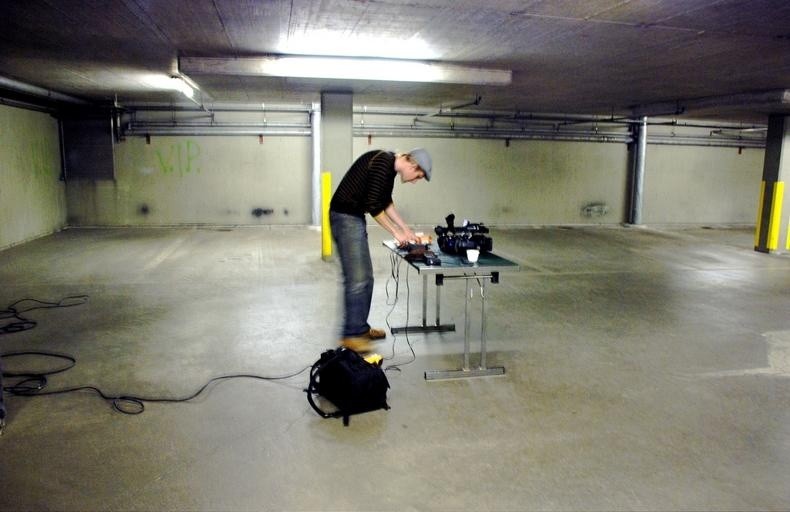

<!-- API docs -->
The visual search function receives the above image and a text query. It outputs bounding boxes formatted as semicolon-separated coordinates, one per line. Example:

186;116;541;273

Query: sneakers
367;328;386;339
341;336;371;353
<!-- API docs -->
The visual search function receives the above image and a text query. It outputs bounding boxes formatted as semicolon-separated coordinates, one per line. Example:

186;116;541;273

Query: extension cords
363;354;383;365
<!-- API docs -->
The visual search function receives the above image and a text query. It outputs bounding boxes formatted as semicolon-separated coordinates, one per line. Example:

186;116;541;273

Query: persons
328;147;432;355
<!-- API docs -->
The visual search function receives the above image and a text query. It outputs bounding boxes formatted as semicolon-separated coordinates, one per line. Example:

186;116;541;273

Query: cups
466;248;480;264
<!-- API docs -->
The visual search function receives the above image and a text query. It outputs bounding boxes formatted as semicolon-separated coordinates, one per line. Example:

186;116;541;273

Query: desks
382;239;518;380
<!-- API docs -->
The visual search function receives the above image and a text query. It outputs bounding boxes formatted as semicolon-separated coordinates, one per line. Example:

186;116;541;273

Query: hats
410;147;433;181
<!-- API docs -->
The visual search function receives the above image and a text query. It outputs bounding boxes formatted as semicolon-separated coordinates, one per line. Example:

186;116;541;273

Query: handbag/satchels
303;346;391;427
